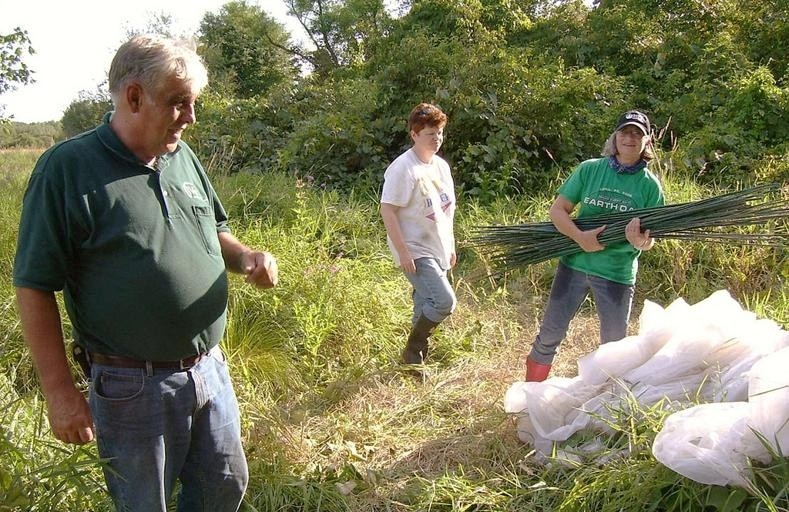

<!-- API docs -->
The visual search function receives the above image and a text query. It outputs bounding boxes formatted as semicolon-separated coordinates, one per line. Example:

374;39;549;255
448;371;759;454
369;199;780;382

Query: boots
402;309;440;365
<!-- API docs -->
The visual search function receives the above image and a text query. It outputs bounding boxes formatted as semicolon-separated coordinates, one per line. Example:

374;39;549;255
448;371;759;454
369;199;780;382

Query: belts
88;350;206;371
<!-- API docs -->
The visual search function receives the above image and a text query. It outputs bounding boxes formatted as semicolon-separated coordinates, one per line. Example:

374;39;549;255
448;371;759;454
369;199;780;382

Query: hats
615;110;651;136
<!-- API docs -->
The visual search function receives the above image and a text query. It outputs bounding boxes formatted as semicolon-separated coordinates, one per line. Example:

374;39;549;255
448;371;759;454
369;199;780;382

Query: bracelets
638;239;648;250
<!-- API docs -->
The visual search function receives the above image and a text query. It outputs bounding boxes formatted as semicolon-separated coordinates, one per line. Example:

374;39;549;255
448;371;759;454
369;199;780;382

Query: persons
11;31;283;512
377;102;463;381
523;107;668;386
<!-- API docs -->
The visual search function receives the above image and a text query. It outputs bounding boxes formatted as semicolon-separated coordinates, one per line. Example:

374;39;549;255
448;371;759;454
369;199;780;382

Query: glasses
616;130;644;139
